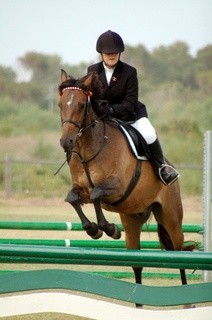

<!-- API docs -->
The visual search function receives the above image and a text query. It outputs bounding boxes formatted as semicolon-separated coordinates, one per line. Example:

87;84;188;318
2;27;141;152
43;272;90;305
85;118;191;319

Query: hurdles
0;220;212;320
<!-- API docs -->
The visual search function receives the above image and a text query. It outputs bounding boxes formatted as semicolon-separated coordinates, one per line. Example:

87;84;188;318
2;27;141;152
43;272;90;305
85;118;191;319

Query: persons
88;29;179;185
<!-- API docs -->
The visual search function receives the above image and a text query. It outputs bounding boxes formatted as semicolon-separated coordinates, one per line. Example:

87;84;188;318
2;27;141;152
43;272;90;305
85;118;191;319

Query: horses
58;69;204;308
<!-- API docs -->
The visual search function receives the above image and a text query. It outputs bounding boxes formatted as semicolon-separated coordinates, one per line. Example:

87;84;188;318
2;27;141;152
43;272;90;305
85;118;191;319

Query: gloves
97;100;113;116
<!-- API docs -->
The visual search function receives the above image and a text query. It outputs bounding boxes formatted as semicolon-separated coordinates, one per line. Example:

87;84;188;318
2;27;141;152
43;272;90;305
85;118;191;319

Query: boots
143;139;180;185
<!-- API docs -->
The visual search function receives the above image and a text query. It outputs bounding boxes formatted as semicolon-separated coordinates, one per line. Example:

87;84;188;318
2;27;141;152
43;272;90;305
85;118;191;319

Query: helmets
96;30;124;53
